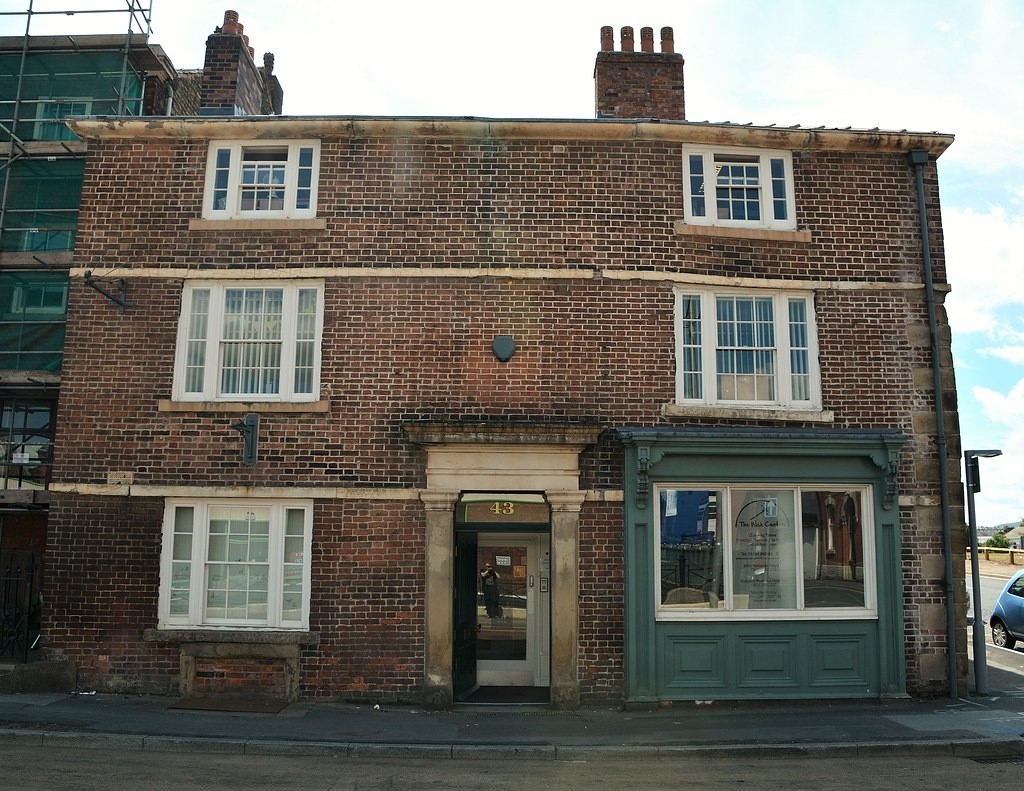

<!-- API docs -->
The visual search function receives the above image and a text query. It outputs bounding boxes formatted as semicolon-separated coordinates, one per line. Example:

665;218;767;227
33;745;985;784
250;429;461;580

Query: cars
988;568;1024;649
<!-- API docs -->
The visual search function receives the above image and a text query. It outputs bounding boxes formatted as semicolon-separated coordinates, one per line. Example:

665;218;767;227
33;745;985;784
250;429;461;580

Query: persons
481;561;503;618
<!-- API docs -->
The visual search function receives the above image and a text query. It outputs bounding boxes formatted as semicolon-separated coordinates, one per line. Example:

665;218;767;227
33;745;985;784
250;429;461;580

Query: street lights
963;449;1003;696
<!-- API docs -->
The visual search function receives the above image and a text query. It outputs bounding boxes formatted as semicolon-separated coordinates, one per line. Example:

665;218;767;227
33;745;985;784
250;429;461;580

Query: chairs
662;587;705;605
733;594;750;609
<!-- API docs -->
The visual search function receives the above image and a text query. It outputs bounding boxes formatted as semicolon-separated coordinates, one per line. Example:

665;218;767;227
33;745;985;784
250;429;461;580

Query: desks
661;601;726;610
